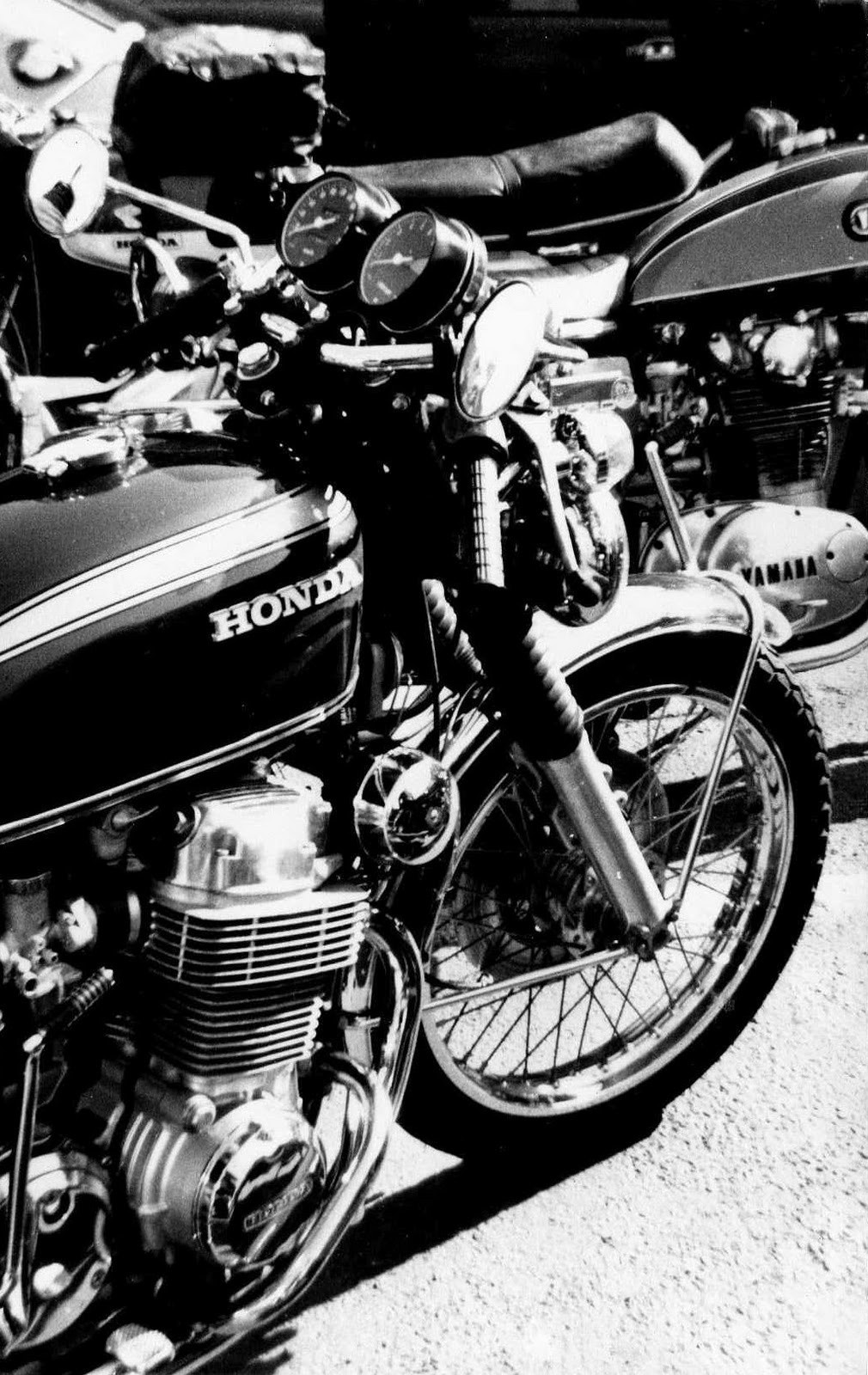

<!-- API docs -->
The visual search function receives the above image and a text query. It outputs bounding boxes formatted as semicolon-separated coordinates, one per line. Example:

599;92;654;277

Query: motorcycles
0;0;868;1375
